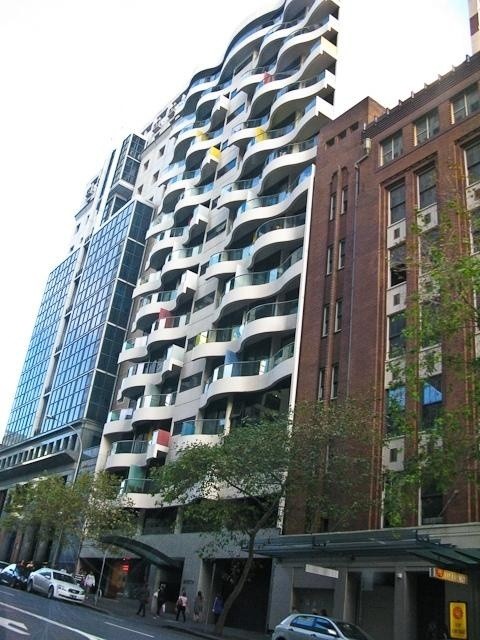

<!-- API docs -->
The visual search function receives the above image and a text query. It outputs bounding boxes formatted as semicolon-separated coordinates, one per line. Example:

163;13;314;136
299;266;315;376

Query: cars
28;568;86;604
271;613;371;640
0;561;30;589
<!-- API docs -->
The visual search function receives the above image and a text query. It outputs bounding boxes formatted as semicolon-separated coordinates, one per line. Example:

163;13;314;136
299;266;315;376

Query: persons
175;592;189;622
153;583;170;618
135;583;152;617
19;559;25;567
192;590;204;623
312;608;319;615
321;608;328;617
292;605;301;615
211;591;224;624
26;560;35;569
75;566;95;600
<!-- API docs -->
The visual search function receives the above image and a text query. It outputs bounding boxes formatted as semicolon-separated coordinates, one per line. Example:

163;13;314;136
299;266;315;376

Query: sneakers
153;615;159;620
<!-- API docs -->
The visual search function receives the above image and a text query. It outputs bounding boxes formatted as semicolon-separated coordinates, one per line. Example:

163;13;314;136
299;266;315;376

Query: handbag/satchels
161;604;166;614
178;599;183;605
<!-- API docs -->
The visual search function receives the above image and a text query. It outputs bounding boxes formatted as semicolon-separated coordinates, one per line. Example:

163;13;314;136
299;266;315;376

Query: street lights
46;414;82;569
